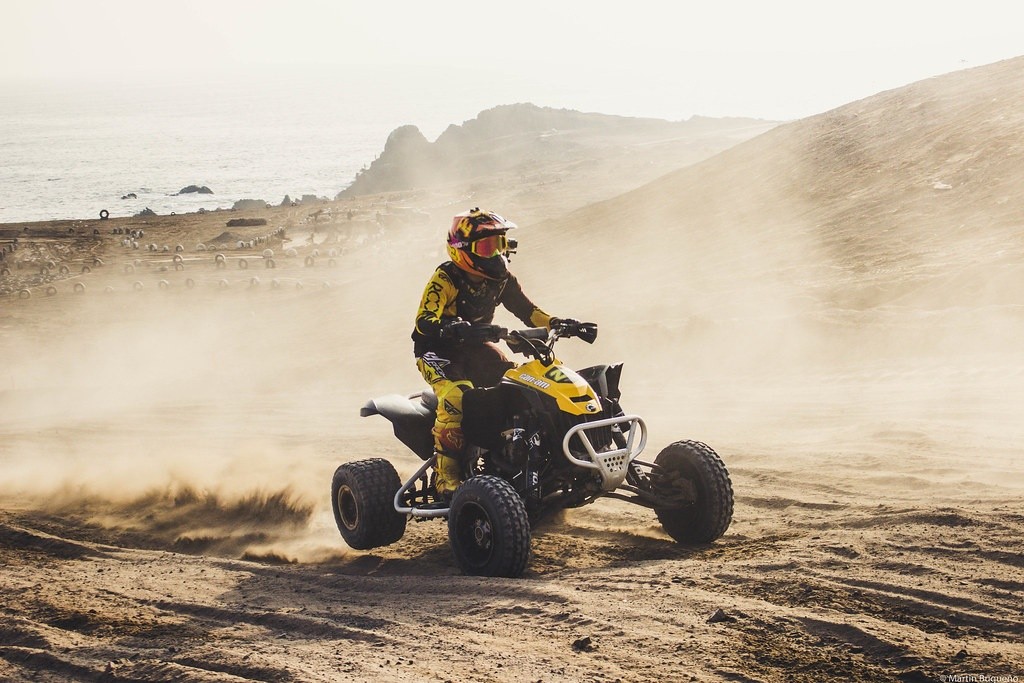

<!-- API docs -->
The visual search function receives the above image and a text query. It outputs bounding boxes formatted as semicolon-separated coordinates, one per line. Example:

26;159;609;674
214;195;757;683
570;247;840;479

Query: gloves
549;316;580;337
441;317;474;343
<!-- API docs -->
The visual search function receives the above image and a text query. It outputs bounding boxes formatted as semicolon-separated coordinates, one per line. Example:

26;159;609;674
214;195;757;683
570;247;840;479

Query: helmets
446;207;517;280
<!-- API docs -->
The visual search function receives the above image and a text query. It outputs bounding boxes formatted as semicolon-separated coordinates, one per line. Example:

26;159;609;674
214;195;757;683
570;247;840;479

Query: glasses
447;231;507;258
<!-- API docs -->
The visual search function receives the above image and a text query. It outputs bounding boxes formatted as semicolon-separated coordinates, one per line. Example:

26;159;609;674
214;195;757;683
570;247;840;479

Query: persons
411;209;584;503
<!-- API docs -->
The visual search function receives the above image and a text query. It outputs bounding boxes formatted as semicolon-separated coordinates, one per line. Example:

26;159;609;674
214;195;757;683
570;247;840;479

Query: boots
432;418;469;500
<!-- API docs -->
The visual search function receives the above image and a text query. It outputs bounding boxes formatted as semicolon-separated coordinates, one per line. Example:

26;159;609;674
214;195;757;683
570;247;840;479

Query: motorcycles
331;320;736;578
